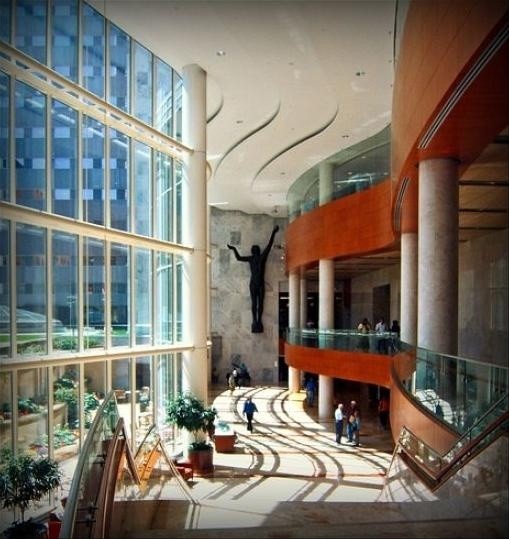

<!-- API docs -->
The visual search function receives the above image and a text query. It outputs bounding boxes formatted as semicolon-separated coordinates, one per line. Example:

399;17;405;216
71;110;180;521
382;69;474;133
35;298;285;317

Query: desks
215;429;237;453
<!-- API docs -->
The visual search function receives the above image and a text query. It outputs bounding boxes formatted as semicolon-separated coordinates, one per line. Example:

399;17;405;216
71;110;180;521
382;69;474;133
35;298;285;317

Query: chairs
172;459;195;484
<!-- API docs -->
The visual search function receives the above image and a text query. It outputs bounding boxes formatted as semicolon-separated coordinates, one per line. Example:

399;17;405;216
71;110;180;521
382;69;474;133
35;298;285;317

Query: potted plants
0;455;62;539
164;389;218;478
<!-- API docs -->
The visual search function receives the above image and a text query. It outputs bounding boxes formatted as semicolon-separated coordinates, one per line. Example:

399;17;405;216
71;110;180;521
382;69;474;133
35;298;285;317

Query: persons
374;317;389;353
335;403;347;445
378;395;389;430
228;372;235;396
306;377;316;407
344;400;357;442
388;320;400;354
242;395;259;433
357;318;372;351
227;225;279;330
348;410;360;447
232;367;241;389
